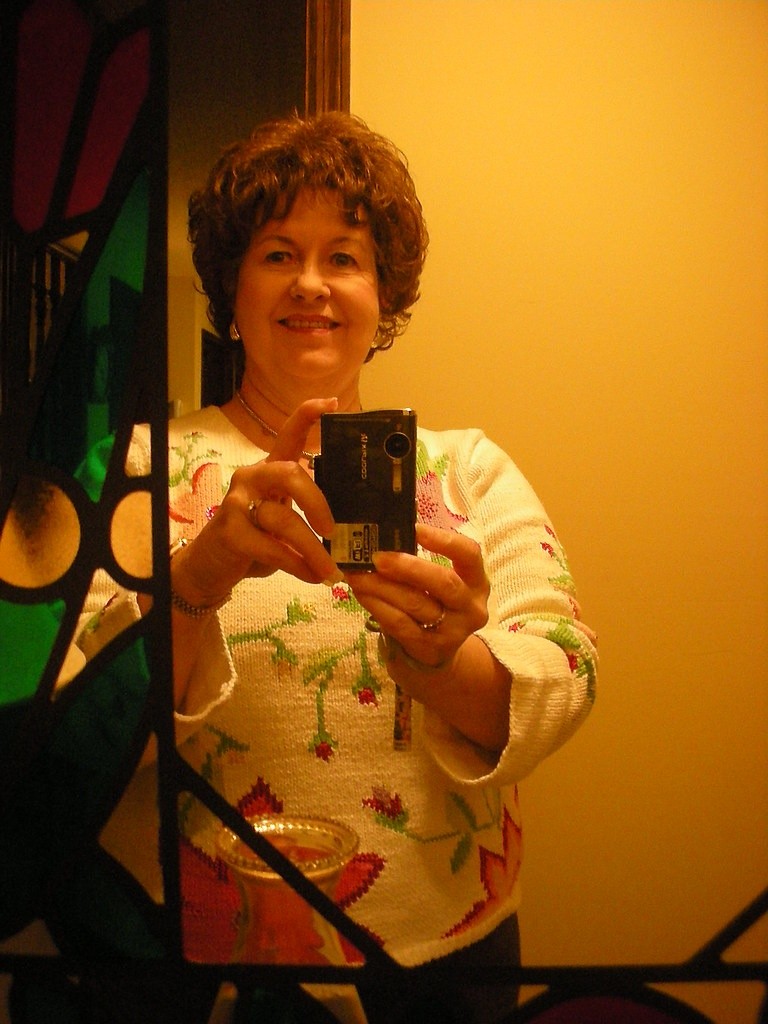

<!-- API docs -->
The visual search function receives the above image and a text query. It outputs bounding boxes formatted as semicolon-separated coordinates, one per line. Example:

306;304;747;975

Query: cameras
319;408;417;572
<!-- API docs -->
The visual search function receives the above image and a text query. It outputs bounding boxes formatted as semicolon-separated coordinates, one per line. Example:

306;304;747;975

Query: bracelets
366;615;452;677
168;538;232;616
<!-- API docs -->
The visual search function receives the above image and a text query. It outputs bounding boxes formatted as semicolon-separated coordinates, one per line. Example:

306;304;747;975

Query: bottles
218;813;370;1024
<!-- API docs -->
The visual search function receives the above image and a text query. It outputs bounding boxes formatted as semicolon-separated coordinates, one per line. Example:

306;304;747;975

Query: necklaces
234;388;363;469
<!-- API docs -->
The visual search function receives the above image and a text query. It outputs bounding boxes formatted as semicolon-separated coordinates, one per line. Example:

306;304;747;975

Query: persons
30;109;596;1024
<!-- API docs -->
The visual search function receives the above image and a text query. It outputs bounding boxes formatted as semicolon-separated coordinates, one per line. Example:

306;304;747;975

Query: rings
418;606;447;629
247;498;266;527
